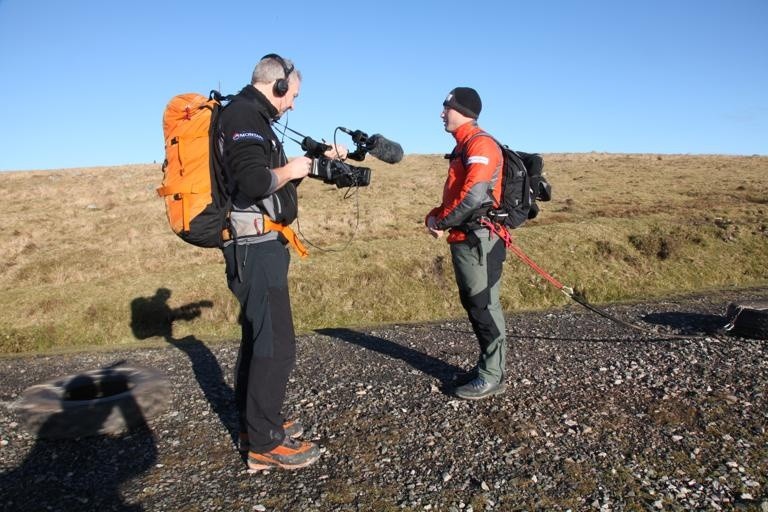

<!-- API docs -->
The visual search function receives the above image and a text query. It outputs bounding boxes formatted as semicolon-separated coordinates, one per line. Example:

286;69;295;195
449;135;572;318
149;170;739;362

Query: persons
423;84;510;401
214;53;353;473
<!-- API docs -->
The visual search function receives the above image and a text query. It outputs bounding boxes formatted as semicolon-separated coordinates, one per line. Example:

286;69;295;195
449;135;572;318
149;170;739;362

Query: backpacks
156;90;272;248
461;131;551;229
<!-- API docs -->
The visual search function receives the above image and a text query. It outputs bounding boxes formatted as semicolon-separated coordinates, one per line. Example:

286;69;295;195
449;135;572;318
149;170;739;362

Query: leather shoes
449;367;479;382
455;376;506;399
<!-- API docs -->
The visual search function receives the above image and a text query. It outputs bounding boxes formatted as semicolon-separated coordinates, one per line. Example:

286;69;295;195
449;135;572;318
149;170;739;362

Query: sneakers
247;434;320;469
238;421;304;450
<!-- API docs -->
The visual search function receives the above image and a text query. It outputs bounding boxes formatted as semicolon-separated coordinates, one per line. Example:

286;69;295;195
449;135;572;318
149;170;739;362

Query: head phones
261;54;295;96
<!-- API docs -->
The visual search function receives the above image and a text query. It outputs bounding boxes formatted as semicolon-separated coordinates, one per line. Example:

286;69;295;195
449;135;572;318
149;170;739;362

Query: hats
444;88;482;120
